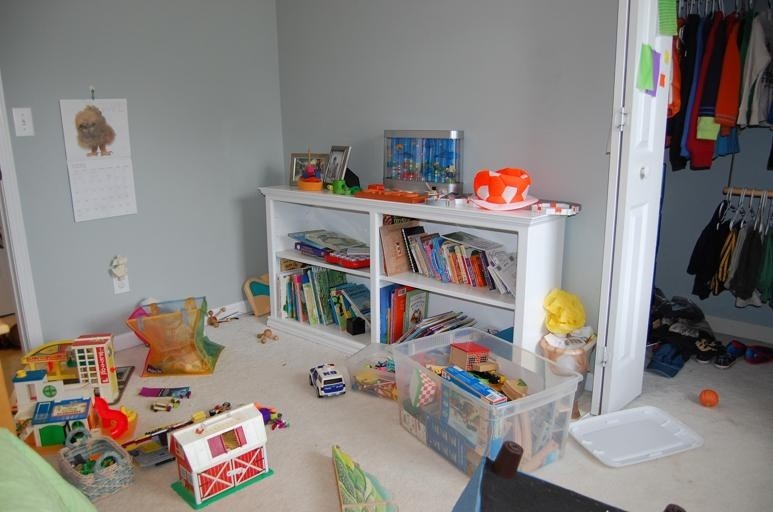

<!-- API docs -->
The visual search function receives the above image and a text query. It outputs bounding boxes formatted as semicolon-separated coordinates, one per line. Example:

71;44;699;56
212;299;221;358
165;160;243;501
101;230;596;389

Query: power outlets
112;275;132;296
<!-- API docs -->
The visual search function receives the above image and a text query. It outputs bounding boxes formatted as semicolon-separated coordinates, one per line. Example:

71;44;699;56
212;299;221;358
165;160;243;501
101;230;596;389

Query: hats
646;342;685;378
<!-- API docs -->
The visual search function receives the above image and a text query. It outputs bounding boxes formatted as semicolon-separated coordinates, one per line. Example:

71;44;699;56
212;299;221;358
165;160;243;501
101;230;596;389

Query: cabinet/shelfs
256;188;566;393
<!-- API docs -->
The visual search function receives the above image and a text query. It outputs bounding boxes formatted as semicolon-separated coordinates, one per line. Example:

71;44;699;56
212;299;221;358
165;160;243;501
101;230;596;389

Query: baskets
58;428;136;504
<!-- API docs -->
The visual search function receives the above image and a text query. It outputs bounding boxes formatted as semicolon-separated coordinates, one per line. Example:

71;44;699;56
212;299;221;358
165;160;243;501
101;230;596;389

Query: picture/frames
323;146;361;189
289;153;330;187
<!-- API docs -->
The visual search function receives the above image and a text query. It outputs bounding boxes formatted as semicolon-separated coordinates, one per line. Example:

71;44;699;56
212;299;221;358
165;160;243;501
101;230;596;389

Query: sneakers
697;349;738;369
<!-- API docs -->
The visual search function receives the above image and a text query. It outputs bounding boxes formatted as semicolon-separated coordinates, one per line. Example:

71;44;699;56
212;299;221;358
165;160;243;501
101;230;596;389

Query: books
275;214;518;361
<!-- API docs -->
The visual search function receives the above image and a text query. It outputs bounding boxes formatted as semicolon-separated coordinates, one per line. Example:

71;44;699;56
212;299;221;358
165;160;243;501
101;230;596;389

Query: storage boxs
389;326;582;482
343;342;400;401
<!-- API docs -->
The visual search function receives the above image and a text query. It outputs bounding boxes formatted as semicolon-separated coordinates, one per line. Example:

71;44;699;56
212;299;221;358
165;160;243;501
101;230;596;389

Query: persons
314;158;323;178
325;156;338;181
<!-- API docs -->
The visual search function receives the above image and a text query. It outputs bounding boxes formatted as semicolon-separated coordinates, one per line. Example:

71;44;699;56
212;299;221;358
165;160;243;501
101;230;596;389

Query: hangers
716;186;773;237
678;0;755;19
